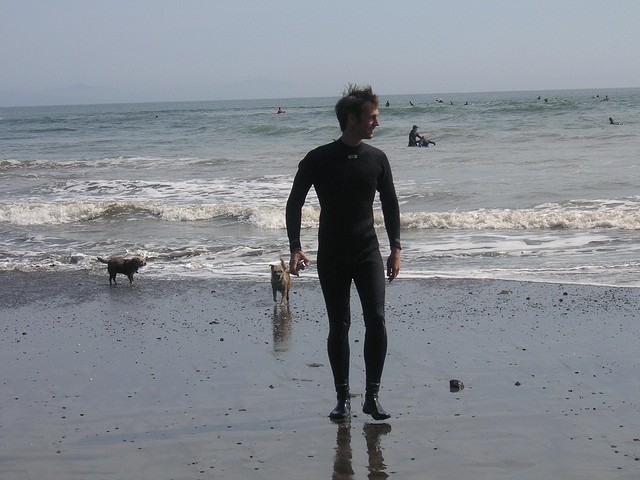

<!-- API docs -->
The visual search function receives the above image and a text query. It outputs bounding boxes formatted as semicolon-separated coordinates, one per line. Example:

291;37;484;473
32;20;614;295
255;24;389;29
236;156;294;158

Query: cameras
297;259;306;270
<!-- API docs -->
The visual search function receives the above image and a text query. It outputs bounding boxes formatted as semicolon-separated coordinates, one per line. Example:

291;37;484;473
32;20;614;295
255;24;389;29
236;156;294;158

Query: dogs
97;256;146;285
269;257;291;306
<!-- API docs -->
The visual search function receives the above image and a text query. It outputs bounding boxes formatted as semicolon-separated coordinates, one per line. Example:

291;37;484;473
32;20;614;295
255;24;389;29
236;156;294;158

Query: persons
416;136;436;147
409;124;421;147
285;86;400;420
277;107;282;113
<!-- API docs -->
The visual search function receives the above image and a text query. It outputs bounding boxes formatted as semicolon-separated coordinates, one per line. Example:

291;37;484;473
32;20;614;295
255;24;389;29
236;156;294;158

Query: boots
330;383;351;419
362;384;391;419
